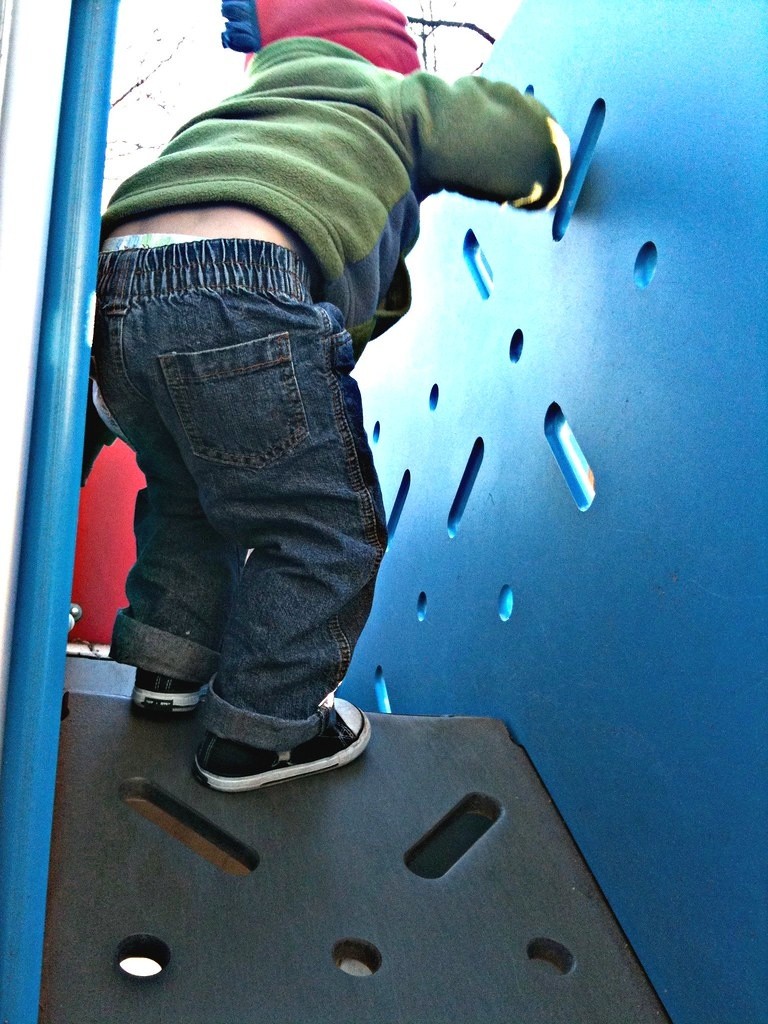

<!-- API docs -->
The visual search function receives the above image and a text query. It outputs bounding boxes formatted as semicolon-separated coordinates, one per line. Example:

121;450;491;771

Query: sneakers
131;665;209;714
193;698;373;793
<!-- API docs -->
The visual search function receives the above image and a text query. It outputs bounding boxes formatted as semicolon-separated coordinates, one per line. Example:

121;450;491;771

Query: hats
223;0;420;76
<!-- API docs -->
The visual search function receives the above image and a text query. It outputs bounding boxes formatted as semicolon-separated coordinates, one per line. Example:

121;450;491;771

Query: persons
91;1;571;792
